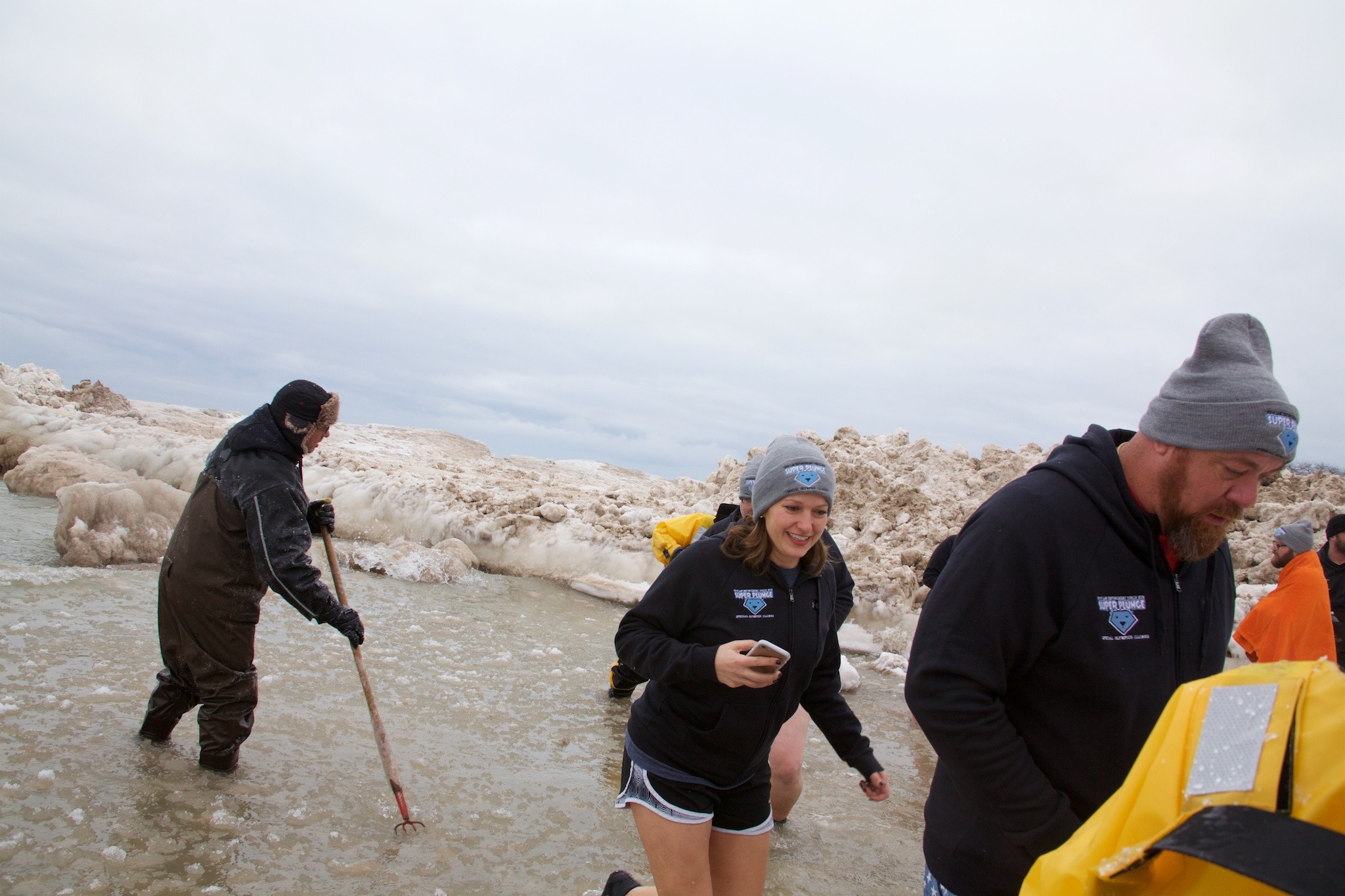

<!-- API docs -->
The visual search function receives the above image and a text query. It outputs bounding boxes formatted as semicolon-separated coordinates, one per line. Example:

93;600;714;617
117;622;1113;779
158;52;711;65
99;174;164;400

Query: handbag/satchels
1020;656;1345;895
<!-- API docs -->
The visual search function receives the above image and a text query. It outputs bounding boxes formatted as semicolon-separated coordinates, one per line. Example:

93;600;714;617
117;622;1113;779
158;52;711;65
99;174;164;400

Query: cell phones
744;639;790;674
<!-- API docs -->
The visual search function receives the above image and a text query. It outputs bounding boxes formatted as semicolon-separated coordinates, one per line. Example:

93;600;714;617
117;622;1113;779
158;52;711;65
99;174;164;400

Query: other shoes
602;871;641;896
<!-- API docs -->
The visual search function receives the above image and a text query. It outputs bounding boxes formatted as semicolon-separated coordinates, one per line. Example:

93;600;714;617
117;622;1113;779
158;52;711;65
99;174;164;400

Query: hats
739;454;766;499
1274;520;1313;553
752;435;836;523
1326;514;1345;540
1140;314;1299;462
269;380;339;454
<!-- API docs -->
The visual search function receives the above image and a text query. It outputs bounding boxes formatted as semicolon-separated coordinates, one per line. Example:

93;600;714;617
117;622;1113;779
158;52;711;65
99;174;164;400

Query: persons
1019;660;1345;896
904;313;1300;896
138;380;364;772
1317;513;1345;670
700;454;855;828
608;504;739;698
1232;519;1337;664
602;435;889;896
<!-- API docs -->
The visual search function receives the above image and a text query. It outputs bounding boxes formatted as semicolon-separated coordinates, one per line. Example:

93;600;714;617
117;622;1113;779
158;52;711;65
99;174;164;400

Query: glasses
315;425;330;433
740;498;752;505
1273;543;1288;550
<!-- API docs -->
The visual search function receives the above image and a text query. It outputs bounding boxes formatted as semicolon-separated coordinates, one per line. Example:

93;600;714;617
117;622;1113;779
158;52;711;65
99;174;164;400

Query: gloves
316;597;364;649
307;499;335;534
